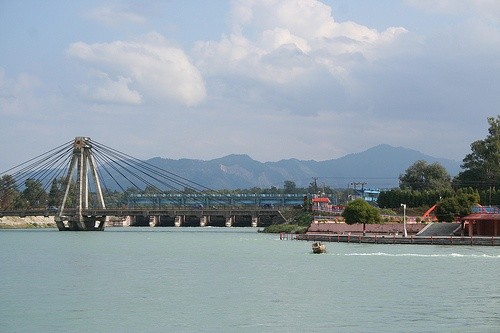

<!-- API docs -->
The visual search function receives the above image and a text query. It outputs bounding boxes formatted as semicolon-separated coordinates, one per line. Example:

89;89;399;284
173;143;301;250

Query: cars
47;206;59;210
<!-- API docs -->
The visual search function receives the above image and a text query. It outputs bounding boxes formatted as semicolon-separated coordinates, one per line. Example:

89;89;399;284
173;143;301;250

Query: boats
312;242;326;253
